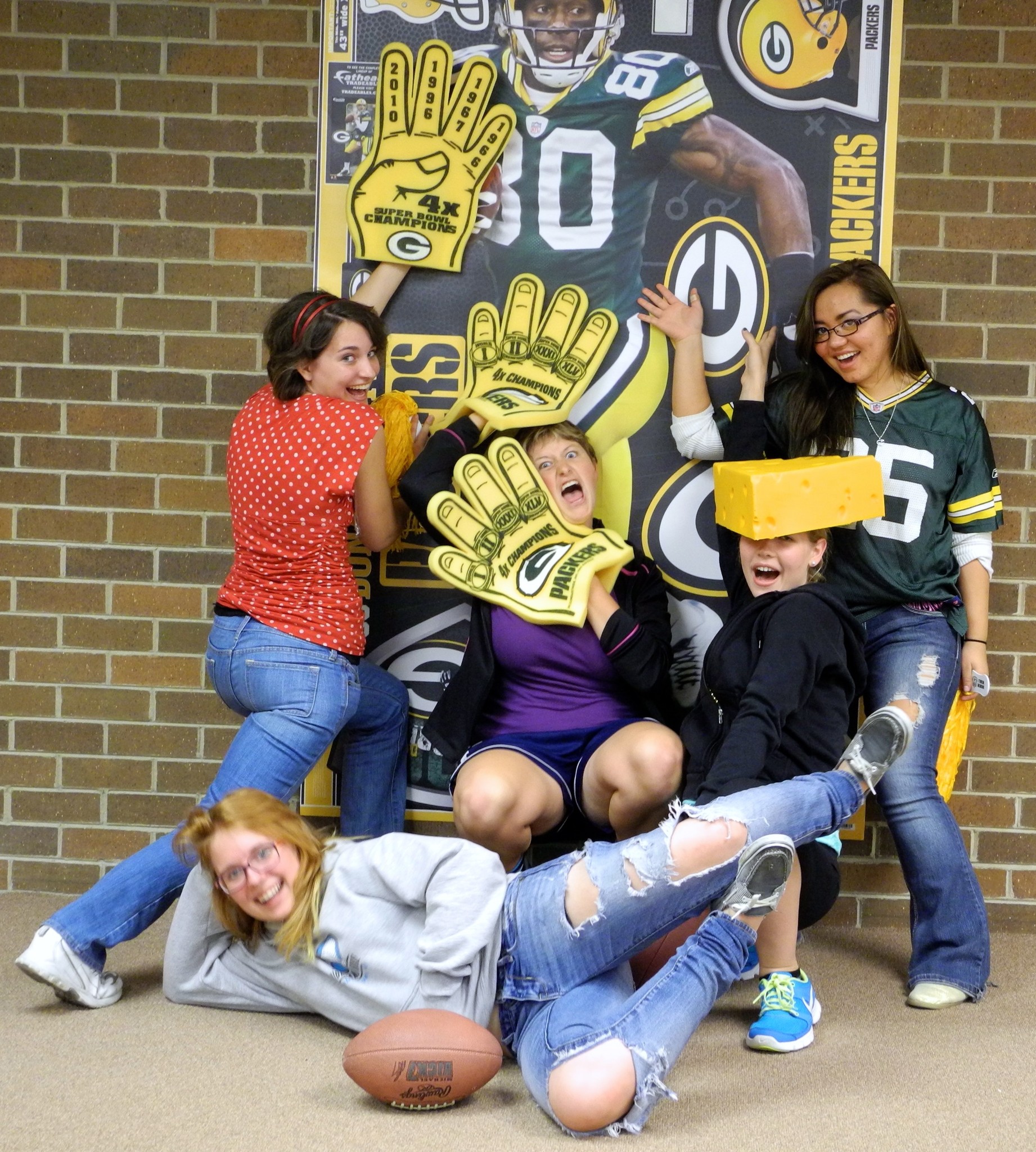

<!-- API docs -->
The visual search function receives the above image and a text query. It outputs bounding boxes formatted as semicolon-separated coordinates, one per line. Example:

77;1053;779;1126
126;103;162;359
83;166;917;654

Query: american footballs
629;903;711;992
340;1009;504;1110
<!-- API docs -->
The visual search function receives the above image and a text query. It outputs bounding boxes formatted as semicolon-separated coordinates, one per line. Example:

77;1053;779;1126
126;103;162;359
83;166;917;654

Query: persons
682;327;864;1053
398;412;684;868
161;705;913;1141
477;0;815;546
640;256;1004;1007
14;263;434;1009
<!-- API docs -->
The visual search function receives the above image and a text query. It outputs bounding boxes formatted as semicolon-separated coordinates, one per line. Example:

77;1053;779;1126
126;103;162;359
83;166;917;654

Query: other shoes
710;834;796;921
907;982;969;1010
832;706;914;802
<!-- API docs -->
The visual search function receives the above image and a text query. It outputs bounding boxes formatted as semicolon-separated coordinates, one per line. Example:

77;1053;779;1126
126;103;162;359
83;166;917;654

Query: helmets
356;98;366;105
499;0;622;69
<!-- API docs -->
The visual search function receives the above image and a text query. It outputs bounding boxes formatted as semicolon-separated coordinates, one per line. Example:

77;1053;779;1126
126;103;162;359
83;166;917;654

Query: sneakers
13;924;123;1009
337;168;350;177
732;946;761;981
745;968;821;1053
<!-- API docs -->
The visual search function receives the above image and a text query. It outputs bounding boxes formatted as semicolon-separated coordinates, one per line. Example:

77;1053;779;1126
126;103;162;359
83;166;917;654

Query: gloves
425;436;635;629
346;41;517;273
768;254;815;370
437;273;620;447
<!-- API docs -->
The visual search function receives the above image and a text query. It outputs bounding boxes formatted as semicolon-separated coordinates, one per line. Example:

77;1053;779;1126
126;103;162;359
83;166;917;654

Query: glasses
213;840;281;896
813;309;883;343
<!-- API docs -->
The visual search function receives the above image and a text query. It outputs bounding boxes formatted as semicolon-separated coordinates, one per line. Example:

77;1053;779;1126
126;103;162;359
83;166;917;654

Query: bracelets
967;639;986;644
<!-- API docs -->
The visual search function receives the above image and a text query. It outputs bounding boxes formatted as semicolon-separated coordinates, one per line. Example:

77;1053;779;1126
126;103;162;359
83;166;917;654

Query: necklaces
857;371;906;445
